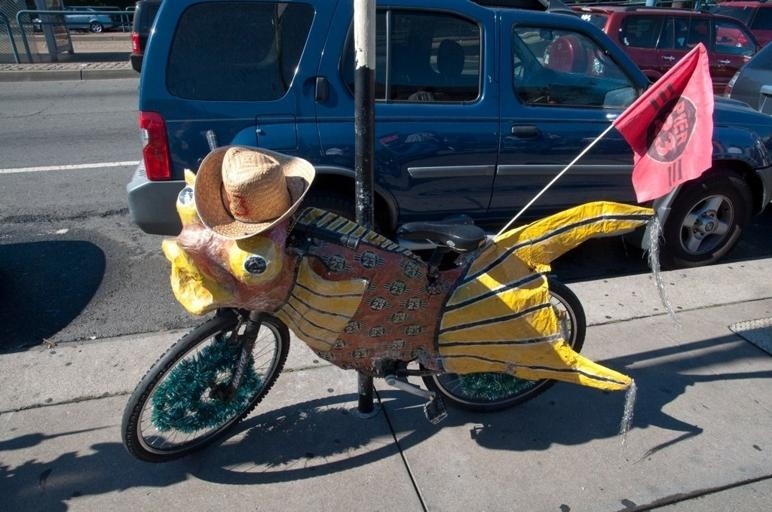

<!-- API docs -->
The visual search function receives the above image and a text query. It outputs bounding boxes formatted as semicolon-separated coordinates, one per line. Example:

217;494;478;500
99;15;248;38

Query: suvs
128;0;168;72
541;1;772;125
124;0;772;271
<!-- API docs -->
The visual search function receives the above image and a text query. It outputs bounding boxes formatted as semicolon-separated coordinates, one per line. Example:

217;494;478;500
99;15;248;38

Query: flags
611;43;716;205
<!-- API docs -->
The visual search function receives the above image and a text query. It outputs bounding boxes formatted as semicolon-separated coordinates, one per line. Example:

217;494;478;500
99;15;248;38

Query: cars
31;5;115;32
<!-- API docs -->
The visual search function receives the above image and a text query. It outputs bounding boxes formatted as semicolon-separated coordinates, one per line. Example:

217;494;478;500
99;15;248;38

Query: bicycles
118;128;589;468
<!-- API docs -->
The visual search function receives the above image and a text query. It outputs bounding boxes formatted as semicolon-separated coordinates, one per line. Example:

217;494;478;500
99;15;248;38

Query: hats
191;142;317;241
